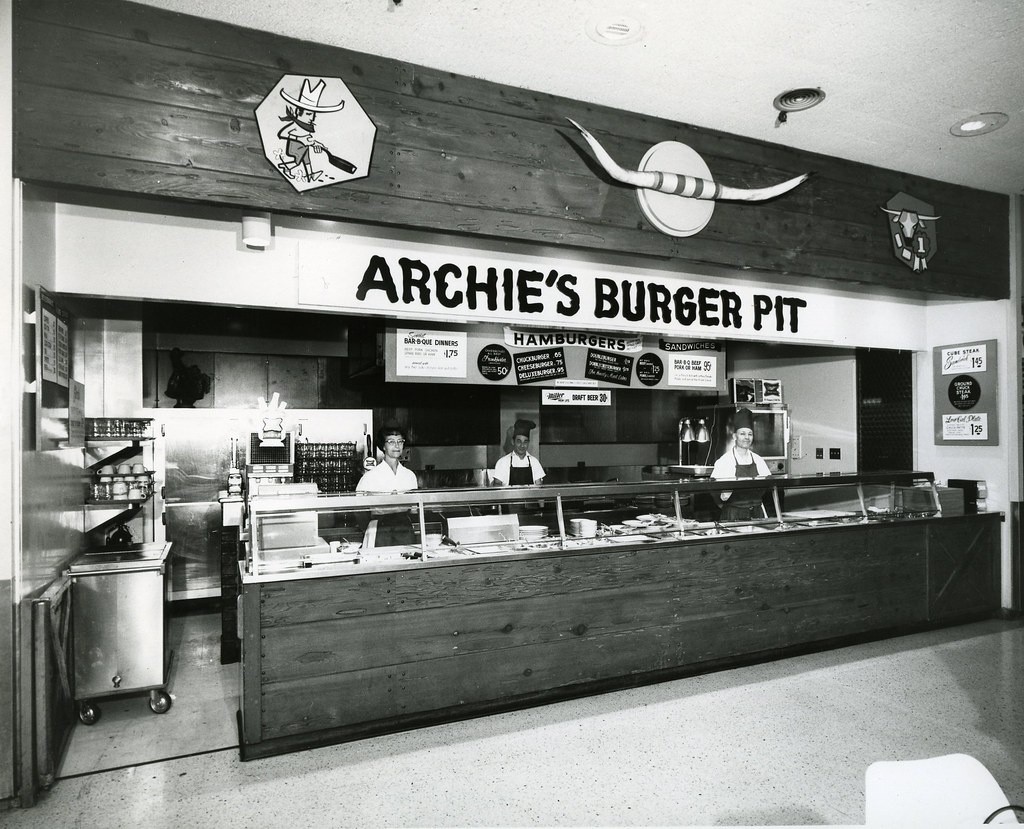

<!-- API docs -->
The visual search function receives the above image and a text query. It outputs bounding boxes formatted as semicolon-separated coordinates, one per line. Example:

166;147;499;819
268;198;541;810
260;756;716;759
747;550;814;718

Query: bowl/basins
425;534;443;547
124;477;135;481
337;542;362;554
100;477;112;482
137;476;149;481
113;477;123;481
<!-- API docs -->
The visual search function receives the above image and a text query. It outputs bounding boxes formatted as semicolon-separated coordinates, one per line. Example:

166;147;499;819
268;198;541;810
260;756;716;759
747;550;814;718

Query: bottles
343;515;348;528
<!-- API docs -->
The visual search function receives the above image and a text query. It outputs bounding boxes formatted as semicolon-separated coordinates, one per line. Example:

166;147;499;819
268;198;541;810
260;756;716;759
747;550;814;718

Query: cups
97;465;114;474
118;465;130;474
128;489;146;499
132;464;148;473
330;541;340;553
113;483;128;495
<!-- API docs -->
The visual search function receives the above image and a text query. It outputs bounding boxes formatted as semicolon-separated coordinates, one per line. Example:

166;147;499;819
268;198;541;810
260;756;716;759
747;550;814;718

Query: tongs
715;521;724;534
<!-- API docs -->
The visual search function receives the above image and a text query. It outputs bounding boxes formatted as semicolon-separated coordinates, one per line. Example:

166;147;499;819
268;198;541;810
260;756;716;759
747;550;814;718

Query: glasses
384;439;404;445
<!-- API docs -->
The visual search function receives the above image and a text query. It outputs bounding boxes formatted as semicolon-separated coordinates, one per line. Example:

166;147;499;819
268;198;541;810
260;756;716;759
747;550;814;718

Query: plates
623;520;646;527
571;519;597;537
519;526;548;540
636;514;667;521
527;543;551;551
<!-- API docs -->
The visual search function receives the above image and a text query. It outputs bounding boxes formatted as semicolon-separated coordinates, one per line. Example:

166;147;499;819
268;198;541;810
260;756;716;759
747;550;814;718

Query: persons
351;425;418;547
491;428;546;526
708;418;772;523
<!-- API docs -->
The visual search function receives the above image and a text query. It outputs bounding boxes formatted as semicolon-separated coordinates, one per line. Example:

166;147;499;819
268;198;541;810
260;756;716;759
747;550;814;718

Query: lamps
241;209;271;247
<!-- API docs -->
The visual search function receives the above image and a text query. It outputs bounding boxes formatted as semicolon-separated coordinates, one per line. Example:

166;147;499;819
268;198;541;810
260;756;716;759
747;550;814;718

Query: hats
732;407;754;431
513;419;536;438
